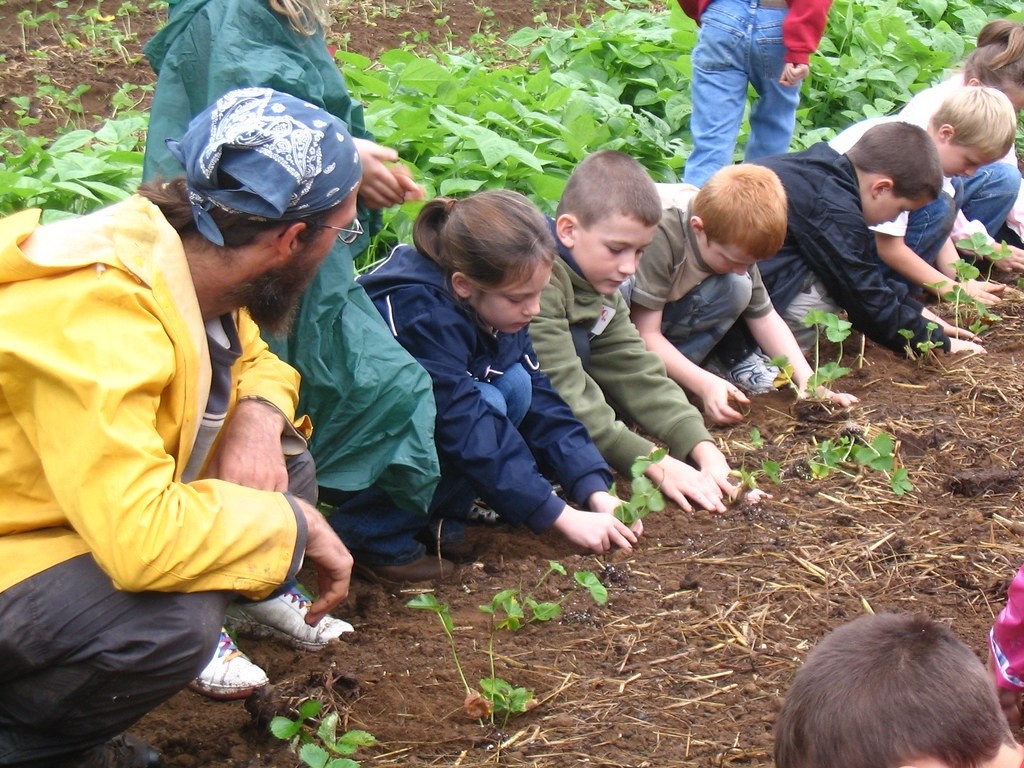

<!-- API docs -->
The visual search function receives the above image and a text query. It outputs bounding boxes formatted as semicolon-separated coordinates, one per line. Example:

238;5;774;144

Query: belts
758;0;790;9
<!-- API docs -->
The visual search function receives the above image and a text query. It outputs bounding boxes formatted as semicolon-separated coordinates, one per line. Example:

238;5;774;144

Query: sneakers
228;586;355;654
464;496;501;524
188;628;271;699
704;345;784;394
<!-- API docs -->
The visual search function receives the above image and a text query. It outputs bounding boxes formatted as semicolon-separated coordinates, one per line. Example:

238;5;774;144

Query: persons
827;85;1024;309
706;120;989;390
142;1;441;699
772;615;1024;768
677;0;832;191
900;17;1024;274
0;84;363;768
615;161;861;429
462;151;773;529
325;190;643;587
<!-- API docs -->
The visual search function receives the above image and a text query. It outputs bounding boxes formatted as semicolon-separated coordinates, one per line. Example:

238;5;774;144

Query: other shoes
45;732;162;768
348;544;455;591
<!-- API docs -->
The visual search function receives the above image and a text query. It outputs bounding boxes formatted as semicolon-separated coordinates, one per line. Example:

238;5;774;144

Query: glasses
321;217;364;244
